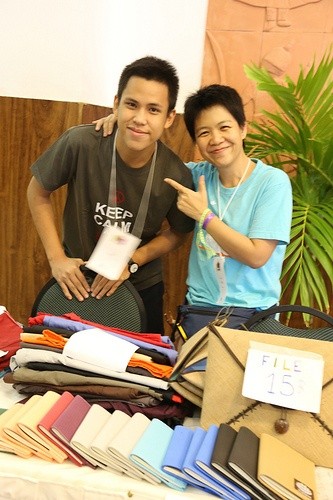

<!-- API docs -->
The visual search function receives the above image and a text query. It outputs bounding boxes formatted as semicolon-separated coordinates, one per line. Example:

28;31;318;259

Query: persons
93;84;293;347
26;57;195;335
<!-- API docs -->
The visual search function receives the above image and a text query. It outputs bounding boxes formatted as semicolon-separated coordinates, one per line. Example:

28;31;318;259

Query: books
0;390;318;500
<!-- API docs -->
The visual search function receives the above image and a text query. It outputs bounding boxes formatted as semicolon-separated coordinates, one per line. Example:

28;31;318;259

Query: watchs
128;258;144;274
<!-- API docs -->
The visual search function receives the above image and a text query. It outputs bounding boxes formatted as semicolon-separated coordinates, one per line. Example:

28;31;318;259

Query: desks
0;452;333;500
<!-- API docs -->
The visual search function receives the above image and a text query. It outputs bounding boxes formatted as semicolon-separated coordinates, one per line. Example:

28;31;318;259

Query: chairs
31;266;147;333
238;305;333;343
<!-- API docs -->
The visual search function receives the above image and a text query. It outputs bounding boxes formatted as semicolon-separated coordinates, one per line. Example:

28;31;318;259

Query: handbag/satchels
172;305;257;342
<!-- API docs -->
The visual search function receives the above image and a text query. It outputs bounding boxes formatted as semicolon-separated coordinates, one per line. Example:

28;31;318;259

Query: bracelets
197;207;216;232
